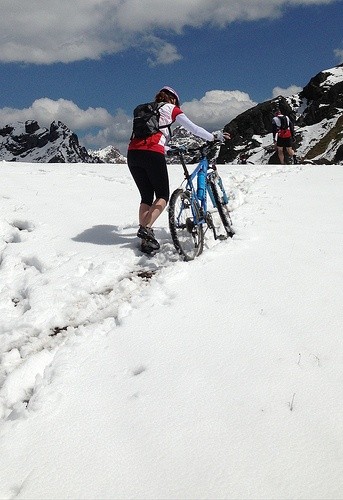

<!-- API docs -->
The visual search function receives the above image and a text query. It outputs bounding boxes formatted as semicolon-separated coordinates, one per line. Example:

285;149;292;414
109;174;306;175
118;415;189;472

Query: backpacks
130;102;175;140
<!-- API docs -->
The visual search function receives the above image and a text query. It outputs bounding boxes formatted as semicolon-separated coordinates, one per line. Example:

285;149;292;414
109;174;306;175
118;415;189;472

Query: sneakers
137;227;160;249
141;240;153;253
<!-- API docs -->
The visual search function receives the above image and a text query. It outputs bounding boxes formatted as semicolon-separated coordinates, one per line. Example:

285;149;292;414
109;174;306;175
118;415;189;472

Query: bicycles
167;139;236;262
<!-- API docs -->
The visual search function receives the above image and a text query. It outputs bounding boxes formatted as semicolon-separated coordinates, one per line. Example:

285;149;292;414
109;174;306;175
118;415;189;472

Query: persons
127;86;230;255
271;107;298;165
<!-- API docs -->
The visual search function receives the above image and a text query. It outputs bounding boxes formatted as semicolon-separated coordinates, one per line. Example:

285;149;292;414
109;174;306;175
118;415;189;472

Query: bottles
197;169;206;199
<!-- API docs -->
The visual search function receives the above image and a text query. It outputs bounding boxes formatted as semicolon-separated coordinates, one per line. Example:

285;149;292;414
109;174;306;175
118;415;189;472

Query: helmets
159;86;179;106
